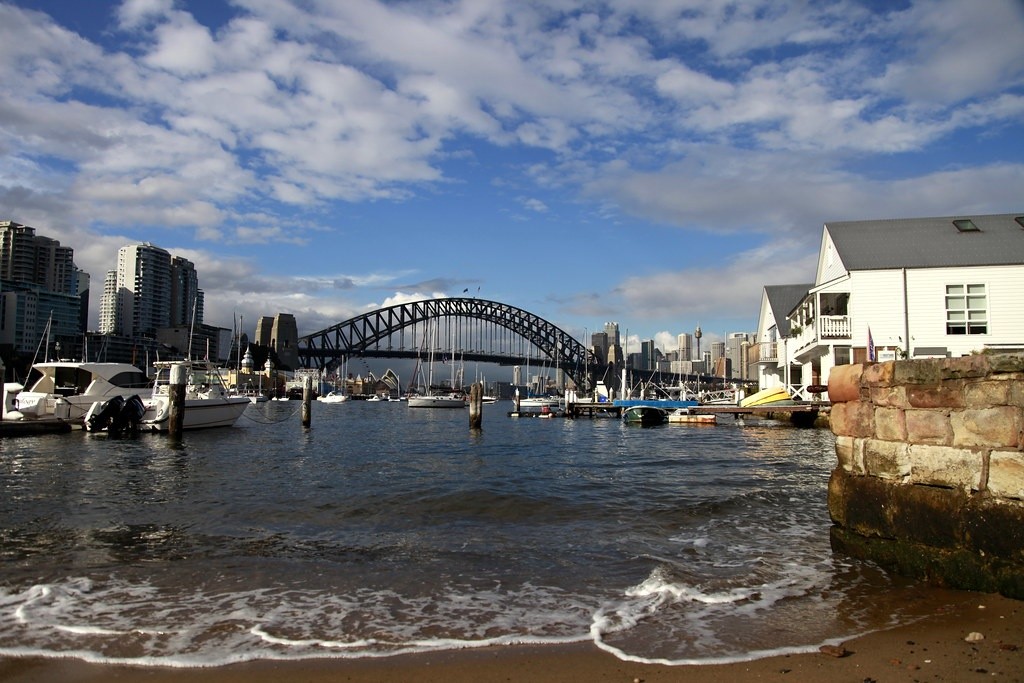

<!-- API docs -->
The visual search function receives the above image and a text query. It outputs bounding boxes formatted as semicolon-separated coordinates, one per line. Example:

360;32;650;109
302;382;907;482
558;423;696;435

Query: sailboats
317;355;353;404
406;317;502;409
509;337;560;407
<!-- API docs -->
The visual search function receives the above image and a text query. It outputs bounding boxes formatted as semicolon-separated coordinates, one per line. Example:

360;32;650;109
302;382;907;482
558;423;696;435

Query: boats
735;385;796;407
668;408;717;422
0;298;291;436
365;393;401;403
625;405;668;427
